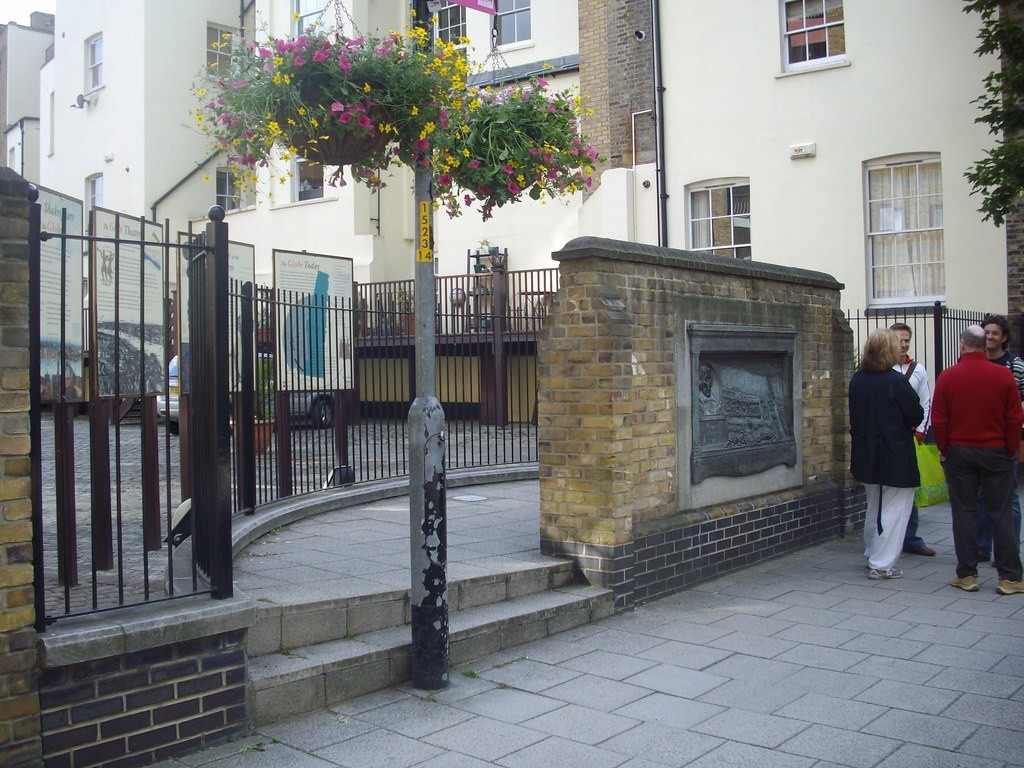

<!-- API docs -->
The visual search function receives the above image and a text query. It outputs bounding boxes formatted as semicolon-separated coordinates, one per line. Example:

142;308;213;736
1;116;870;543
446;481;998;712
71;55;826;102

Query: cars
157;352;335;435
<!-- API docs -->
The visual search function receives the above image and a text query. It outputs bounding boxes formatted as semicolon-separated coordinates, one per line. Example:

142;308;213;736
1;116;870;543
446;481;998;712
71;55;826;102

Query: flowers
191;10;607;221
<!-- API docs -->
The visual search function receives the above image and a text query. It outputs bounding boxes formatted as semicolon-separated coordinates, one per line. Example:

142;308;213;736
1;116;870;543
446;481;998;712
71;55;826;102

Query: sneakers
948;575;980;592
914;546;937;556
866;557;903;579
995;578;1024;595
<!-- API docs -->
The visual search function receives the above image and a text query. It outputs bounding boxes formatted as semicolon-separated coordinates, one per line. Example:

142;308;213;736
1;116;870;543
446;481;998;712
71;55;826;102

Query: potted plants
258;306;273;343
230;359;276;455
400;290;416;335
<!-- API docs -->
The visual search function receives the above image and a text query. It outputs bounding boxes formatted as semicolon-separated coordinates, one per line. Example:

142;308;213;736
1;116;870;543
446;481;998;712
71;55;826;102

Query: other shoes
978;552;990;561
991;561;996;567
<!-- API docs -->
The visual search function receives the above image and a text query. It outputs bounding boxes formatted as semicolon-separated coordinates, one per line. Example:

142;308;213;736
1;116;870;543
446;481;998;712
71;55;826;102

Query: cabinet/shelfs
466;247;509;332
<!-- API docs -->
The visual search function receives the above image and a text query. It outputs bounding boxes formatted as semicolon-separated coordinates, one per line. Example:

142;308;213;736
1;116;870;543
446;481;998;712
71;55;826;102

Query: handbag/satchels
913;436;950;508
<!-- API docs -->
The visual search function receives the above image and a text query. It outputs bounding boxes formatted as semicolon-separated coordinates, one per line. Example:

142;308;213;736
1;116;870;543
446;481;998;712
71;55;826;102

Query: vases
275;103;389;165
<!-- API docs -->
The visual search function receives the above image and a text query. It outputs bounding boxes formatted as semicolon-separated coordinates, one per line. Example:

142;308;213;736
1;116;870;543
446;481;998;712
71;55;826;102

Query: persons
931;325;1024;595
848;329;924;579
877;323;937;556
971;315;1024;567
